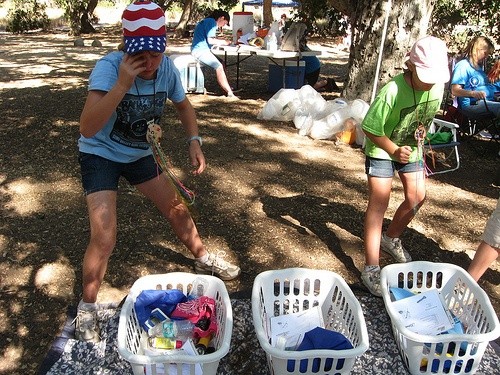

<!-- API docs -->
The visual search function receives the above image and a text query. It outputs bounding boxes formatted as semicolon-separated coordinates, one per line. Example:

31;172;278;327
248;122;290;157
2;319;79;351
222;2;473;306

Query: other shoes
448;286;474;308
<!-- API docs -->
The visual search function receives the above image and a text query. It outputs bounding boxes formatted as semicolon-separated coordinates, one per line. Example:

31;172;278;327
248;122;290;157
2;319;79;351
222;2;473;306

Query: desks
212;46;320;97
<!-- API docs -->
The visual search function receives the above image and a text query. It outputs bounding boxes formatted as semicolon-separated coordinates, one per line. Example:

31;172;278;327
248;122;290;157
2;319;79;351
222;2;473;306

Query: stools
170;54;204;93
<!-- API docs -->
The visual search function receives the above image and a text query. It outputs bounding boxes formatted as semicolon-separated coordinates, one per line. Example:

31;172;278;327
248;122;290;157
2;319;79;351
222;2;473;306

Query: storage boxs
268;61;304;95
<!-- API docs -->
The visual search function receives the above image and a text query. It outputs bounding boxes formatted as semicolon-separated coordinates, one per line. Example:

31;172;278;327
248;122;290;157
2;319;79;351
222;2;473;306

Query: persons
451;35;500;139
467;197;500;283
361;36;451;298
190;9;239;98
70;2;241;340
280;14;292;35
301;45;340;93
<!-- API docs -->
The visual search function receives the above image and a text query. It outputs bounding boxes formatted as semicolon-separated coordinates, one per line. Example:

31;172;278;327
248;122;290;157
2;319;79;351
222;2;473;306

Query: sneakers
381;234;412;262
194;250;241;280
71;303;103;342
361;269;383;297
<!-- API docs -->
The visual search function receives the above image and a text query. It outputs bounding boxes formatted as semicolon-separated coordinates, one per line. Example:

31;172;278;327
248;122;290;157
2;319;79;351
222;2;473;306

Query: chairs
423;119;460;177
443;60;500;150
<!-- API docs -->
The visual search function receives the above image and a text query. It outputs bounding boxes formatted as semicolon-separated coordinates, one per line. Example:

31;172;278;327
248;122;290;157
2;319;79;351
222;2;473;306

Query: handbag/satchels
424;131;453;145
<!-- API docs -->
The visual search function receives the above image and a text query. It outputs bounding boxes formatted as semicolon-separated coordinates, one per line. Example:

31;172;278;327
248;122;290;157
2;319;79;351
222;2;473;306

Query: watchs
189;136;203;147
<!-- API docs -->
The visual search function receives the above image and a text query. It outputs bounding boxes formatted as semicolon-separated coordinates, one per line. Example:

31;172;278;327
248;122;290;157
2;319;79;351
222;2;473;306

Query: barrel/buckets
233;11;254;45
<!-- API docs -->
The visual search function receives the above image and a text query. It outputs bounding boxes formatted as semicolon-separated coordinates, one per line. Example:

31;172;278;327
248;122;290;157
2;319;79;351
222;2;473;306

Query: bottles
148;337;182;350
271;32;277;51
237;28;243;44
267;35;274;51
148;319;193;338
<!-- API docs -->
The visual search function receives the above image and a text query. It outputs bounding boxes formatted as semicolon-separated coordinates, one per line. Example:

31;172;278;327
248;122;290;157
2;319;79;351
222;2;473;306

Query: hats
121;2;166;54
410;36;451;84
